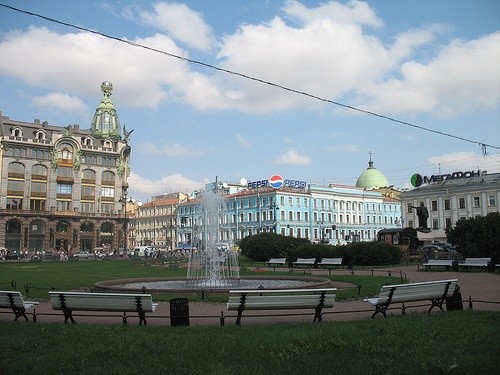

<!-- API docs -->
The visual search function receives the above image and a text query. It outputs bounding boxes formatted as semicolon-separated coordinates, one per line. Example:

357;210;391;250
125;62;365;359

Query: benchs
0;290;39;320
225;288;338;324
364;278;458;316
292;258;317;268
458;257;492;272
265;258;287;268
423;259;456;270
318;256;343;269
210;257;227;266
48;290;159;327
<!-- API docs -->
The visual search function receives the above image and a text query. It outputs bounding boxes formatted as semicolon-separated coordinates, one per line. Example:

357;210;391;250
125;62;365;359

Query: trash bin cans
446;284;463;312
170;297;190;327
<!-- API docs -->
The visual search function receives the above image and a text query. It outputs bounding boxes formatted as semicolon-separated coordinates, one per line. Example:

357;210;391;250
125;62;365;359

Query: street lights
123;183;129;252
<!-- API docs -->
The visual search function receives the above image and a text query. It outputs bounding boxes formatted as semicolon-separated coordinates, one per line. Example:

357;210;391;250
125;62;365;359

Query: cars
402;253;422;262
72;250;95;259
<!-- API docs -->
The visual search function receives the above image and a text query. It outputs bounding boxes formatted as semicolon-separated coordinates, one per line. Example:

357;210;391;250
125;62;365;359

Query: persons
0;245;191;262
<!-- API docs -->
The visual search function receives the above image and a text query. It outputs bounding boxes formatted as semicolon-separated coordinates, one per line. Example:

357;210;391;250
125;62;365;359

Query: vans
131;246;153;257
107;248;131;257
